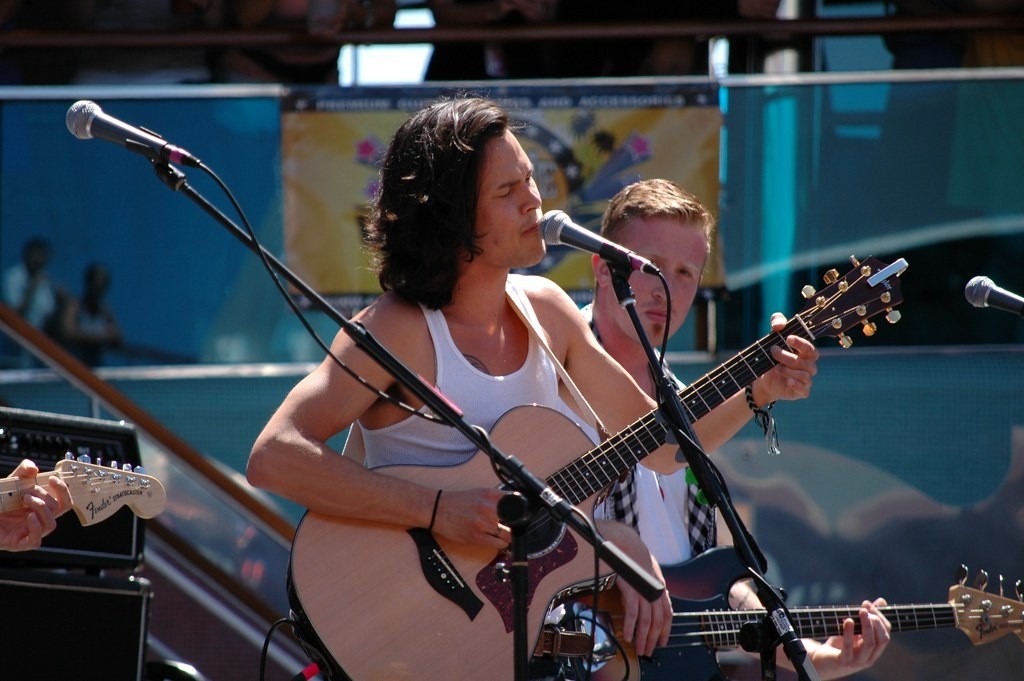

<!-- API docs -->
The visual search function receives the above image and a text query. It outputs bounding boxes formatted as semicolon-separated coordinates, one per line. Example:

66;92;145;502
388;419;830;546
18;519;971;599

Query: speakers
0;562;151;681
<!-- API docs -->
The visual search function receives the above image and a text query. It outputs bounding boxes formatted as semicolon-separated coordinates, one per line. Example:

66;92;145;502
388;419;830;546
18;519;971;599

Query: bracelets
428;489;442;532
745;384;782;457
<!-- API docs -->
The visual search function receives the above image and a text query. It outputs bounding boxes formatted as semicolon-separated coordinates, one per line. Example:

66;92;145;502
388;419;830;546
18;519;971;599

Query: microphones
538;209;661;277
65;99;202;169
964;275;1024;317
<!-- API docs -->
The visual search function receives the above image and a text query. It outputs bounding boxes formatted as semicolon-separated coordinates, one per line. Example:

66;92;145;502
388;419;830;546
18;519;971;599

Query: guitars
526;562;1024;681
287;252;908;681
0;452;168;530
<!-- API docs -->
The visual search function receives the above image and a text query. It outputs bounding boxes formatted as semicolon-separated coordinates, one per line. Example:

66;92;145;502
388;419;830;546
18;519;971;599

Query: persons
247;102;819;681
561;178;891;680
3;234;126;367
0;458;74;551
